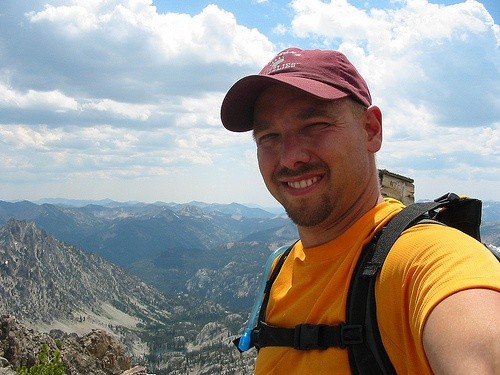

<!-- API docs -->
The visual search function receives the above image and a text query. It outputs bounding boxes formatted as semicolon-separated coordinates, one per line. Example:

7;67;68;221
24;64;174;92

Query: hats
221;48;372;131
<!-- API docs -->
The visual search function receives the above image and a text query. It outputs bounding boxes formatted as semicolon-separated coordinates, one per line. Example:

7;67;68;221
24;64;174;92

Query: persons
220;47;500;375
